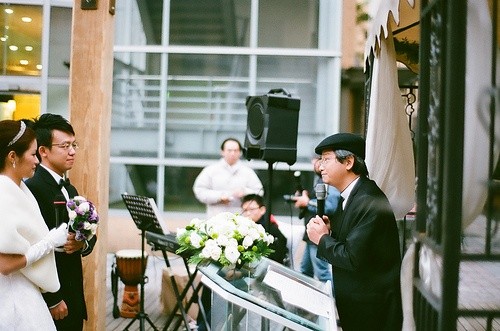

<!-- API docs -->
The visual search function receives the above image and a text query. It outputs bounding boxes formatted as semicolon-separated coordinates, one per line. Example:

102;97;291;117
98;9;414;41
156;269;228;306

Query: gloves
24;223;69;268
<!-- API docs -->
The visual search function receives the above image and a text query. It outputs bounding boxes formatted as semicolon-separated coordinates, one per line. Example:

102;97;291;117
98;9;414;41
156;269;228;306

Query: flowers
175;211;275;271
66;195;99;242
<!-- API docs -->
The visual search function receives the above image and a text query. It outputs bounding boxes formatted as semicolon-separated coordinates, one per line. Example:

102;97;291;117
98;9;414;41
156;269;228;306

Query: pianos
146;229;218;331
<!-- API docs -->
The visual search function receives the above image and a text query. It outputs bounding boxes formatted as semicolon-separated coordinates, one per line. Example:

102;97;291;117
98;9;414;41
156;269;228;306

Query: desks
161;265;203;320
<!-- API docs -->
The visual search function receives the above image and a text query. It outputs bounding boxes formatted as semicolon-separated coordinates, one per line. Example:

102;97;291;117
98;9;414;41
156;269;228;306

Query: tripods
120;193;166;331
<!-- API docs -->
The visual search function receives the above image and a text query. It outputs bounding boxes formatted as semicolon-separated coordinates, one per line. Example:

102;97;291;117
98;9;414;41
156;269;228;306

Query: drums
115;249;148;318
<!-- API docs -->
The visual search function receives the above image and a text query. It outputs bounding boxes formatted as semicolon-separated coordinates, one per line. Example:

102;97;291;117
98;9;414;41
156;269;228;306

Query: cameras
283;171;304;203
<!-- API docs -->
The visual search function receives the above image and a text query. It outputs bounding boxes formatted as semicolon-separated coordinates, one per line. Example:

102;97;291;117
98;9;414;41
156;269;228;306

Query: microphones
315;184;327;219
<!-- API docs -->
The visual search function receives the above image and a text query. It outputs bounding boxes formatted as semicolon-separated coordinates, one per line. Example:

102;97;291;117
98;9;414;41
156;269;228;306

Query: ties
332;195;345;237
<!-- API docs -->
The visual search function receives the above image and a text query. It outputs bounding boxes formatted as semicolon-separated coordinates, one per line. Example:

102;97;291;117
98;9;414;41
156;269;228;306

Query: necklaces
340;175;360;194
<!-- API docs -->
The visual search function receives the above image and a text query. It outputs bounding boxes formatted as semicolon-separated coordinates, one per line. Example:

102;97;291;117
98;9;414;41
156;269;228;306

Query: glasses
51;139;79;151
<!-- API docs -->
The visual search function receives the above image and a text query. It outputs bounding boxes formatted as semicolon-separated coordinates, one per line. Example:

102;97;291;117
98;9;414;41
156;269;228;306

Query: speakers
242;95;301;166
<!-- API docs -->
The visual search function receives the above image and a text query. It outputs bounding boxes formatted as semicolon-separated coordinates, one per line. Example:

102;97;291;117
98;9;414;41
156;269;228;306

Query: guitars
296;172;307;218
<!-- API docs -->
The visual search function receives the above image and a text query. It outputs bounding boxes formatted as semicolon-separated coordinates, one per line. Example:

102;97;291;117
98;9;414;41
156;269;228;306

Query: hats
314;132;366;161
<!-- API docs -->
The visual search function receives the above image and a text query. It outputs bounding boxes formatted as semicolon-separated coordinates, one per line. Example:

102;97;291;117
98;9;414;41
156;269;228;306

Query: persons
291;155;341;298
181;194;289;331
0;120;70;331
304;133;404;331
192;138;264;219
23;113;98;331
